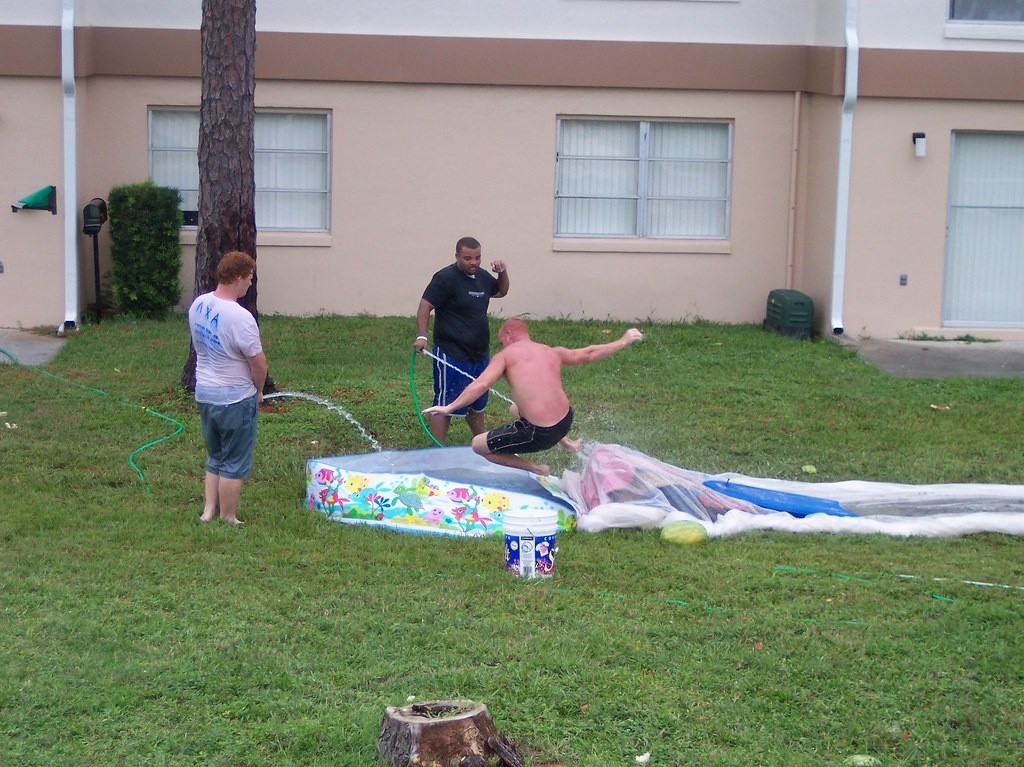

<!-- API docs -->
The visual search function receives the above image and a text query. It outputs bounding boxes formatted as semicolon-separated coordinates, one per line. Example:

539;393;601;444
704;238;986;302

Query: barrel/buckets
502;510;560;578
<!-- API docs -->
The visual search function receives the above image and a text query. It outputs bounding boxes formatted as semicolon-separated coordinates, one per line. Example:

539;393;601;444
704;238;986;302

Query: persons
188;250;268;524
420;318;643;477
413;236;509;450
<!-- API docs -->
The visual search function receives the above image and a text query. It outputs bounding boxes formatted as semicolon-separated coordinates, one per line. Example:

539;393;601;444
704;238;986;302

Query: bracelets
417;336;428;341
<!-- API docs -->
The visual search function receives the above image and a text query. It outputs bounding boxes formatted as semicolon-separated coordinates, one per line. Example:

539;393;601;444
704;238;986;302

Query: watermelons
659;520;709;544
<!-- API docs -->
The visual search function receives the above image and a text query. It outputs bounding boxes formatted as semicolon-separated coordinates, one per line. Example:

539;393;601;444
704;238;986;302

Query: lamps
913;132;926;158
